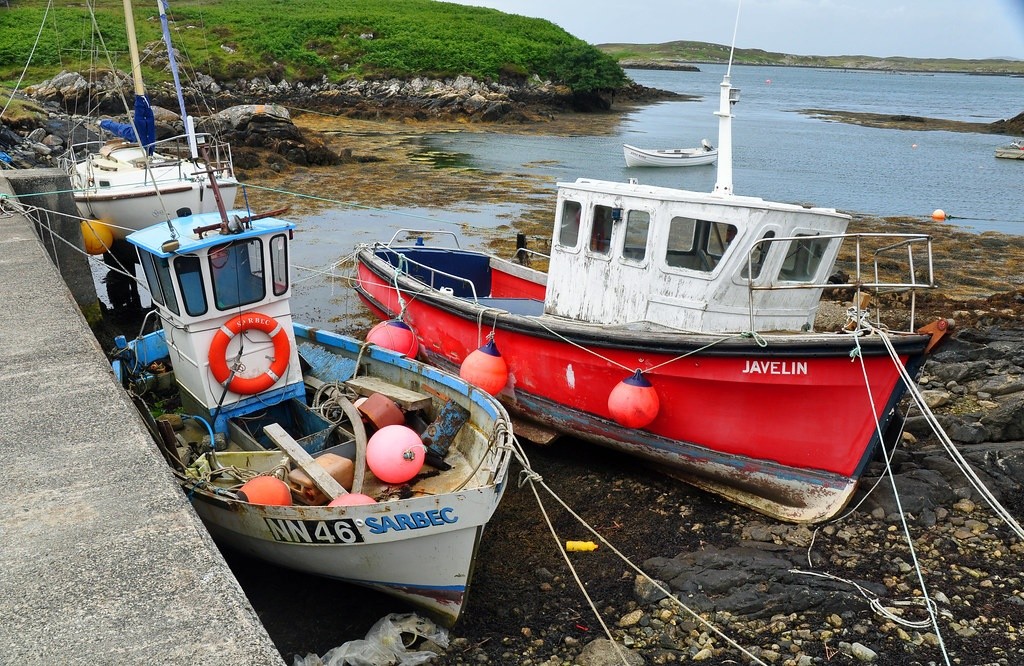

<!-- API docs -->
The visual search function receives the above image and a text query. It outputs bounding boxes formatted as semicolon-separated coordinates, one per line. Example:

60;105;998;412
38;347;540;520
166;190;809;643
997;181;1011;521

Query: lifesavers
209;311;291;394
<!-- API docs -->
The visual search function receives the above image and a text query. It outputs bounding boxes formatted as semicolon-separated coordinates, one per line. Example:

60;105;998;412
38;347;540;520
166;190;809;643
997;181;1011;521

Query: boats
623;138;719;169
112;1;514;626
67;1;238;257
349;0;957;525
